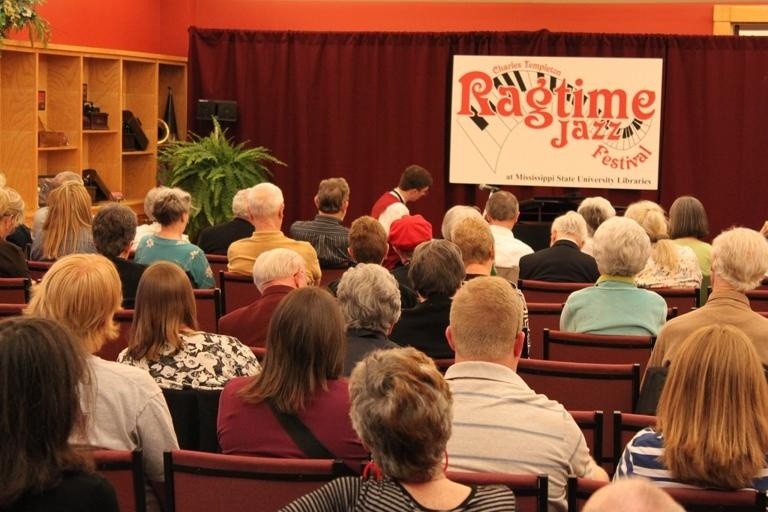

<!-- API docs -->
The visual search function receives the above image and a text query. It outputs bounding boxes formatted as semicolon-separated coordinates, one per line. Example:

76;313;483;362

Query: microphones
479;183;500;191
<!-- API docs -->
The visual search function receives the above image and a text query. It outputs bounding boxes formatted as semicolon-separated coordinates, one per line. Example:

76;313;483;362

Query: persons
640;227;768;393
278;349;519;512
612;322;768;488
330;166;712;375
217;285;371;475
1;165;351;310
440;276;610;511
115;262;263;389
582;476;686;512
22;255;180;510
1;315;119;511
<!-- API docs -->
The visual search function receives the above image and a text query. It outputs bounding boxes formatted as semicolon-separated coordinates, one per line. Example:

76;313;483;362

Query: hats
388;214;432;252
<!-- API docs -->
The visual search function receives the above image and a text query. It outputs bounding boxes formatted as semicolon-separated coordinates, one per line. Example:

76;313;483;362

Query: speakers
218;100;238;120
197;100;216;118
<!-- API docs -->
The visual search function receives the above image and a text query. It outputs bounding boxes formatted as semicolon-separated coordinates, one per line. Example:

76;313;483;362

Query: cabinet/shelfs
0;37;188;229
